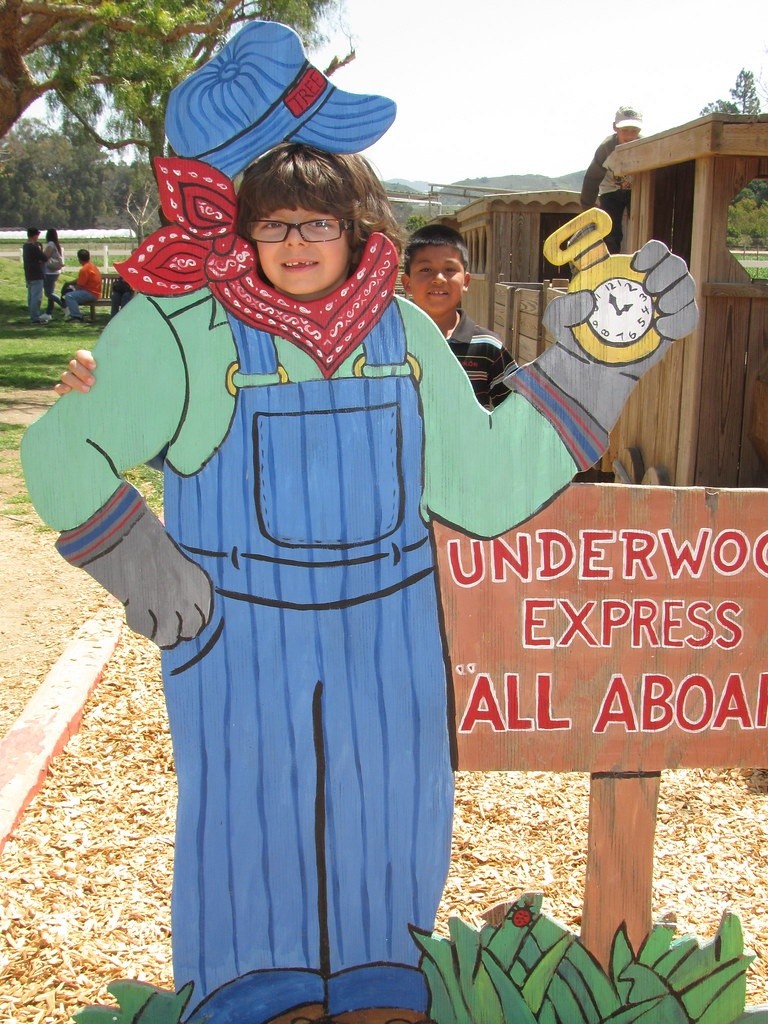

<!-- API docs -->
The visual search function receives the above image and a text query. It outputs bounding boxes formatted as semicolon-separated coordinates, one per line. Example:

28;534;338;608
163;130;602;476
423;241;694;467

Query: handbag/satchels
47;255;64;272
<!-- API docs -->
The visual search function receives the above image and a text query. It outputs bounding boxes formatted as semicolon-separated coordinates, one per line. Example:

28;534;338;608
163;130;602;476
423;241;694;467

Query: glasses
244;214;350;245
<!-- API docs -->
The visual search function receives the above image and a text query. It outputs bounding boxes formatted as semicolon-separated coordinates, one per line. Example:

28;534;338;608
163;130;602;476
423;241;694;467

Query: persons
39;228;70;320
579;104;647;255
400;223;525;413
55;141;408;395
64;248;102;322
22;228;49;324
110;275;134;320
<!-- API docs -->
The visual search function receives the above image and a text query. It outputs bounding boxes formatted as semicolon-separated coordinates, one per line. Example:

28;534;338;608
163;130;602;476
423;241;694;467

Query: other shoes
65;316;84;325
61;306;72;318
39;313;53;320
30;319;49;326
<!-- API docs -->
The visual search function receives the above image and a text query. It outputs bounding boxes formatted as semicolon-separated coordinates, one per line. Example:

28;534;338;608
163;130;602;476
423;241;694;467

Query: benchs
80;274;121;323
